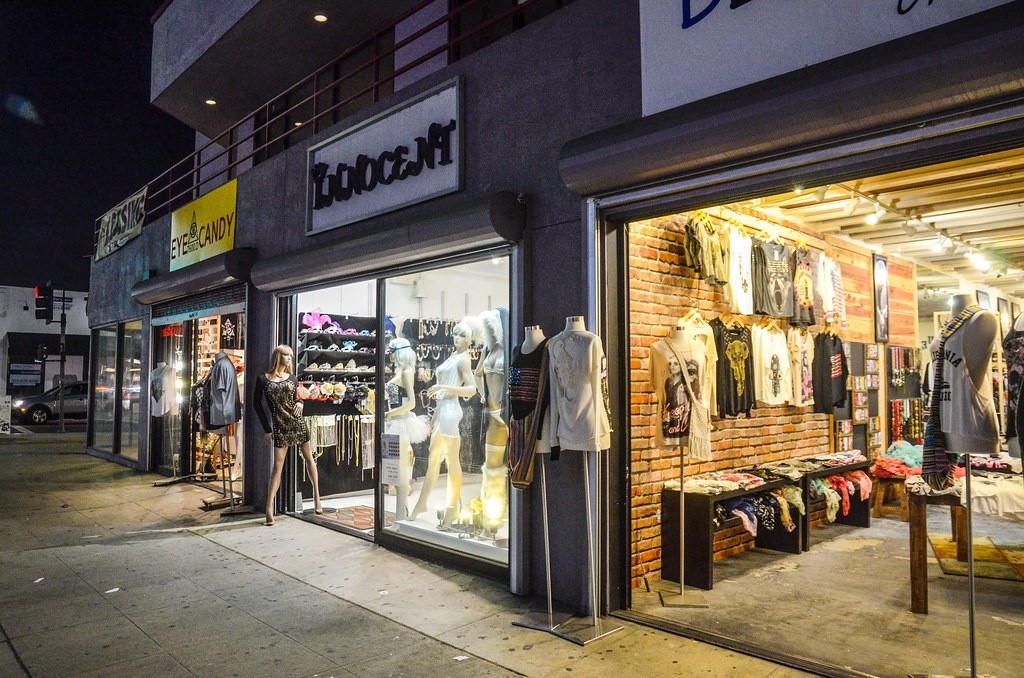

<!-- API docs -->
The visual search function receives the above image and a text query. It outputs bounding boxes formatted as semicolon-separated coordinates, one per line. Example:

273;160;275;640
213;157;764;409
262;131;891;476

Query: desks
661;460;874;590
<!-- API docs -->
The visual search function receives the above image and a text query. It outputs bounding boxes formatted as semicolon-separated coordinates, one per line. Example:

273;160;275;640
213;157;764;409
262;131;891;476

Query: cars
11;381;88;425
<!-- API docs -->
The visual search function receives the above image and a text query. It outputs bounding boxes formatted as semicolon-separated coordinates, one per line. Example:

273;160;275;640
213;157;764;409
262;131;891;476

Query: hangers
824;243;838;262
753;223;783;246
726;212;747;235
823;317;836;335
757;314;781;333
685;300;706;326
791;234;815;262
719;302;745;331
694;210;714;231
794;326;806;332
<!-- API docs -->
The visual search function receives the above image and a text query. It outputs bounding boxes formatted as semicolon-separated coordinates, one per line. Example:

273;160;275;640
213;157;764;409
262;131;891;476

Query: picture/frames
873;253;890;343
977;290;990;311
1011;302;1021;320
998;298;1011;341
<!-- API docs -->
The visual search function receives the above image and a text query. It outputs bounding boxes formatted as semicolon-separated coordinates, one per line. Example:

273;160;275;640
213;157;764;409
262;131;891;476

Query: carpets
317;504;396;530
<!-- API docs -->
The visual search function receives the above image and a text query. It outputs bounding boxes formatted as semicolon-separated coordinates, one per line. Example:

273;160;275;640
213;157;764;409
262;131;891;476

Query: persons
509;325;551;453
929;293;1001;455
384;338;417;532
151;362;178;418
253;344;323;525
546;315;610;451
407;322;476;531
191;351;241;435
474;308;509;541
649;324;704;452
1002;311;1024;458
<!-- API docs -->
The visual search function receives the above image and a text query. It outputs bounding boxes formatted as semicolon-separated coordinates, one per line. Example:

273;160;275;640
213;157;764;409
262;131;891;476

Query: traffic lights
35;280;53;319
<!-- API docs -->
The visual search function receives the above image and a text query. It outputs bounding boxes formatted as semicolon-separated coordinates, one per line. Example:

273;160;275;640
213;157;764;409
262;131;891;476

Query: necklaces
275;372;284;378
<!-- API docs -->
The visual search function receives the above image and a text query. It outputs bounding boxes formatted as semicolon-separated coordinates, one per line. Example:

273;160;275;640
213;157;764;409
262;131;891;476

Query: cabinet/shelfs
195;319;242;465
298;327;394;385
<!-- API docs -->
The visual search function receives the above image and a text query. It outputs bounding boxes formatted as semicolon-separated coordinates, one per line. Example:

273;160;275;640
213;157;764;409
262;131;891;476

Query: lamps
812;184;831;202
899;218;917;237
843;193;861;215
943;239;958;257
864;205;890;225
